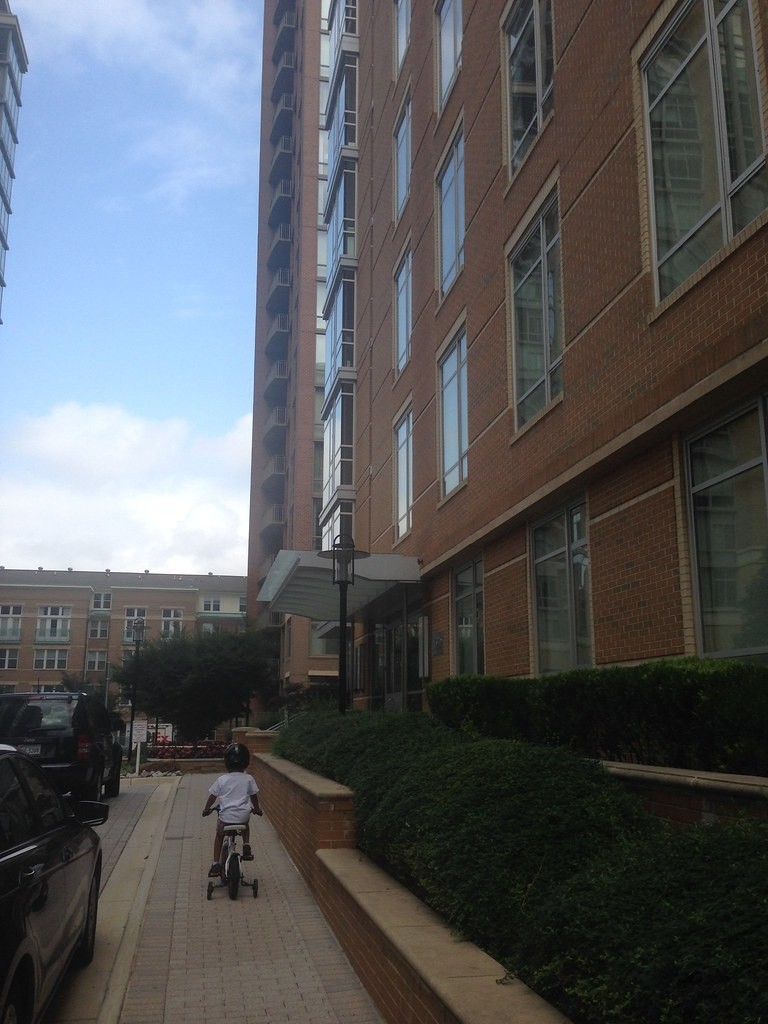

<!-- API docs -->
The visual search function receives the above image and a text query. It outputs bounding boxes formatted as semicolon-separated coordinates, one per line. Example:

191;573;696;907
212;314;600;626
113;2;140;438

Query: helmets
224;742;251;771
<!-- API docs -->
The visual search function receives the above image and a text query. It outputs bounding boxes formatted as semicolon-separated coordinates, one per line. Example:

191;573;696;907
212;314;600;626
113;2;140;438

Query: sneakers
208;864;220;878
243;845;251;857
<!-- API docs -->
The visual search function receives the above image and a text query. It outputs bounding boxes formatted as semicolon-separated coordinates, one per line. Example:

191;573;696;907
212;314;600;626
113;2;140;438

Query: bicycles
201;805;265;901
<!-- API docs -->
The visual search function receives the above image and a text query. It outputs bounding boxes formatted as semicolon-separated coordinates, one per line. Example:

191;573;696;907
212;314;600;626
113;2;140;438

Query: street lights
127;617;152;761
317;535;372;718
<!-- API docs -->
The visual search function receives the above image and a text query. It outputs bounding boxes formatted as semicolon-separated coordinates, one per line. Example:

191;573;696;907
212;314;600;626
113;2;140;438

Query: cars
1;742;110;1024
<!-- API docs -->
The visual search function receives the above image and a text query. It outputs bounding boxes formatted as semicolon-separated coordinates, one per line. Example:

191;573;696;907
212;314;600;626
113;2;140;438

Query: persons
199;742;264;878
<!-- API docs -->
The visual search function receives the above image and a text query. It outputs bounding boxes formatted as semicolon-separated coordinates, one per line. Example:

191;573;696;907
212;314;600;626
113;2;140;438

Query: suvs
0;691;126;807
